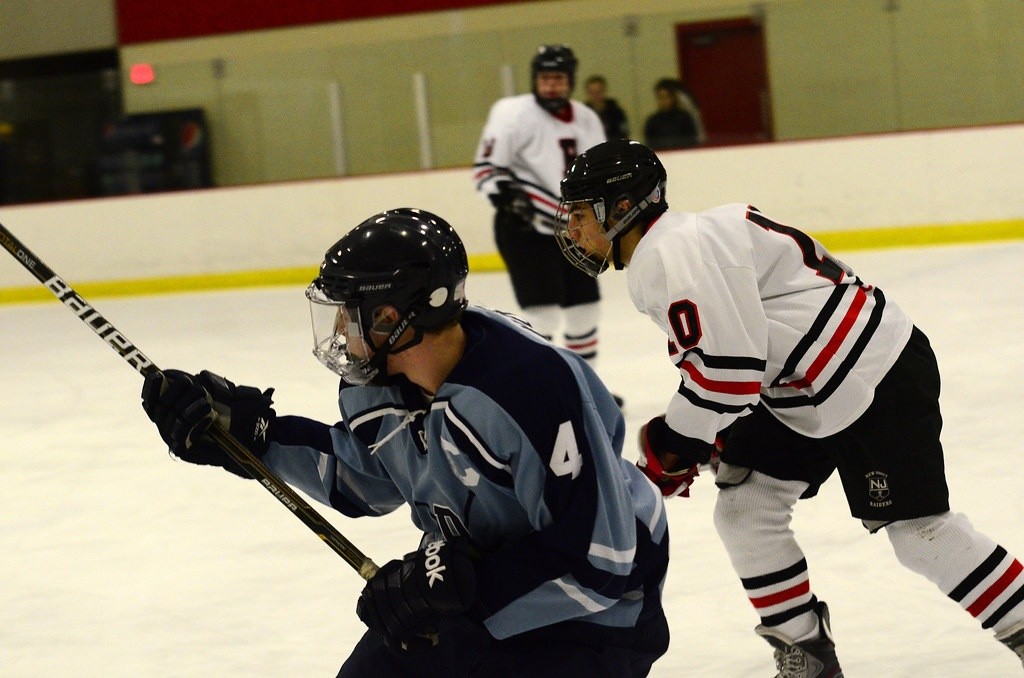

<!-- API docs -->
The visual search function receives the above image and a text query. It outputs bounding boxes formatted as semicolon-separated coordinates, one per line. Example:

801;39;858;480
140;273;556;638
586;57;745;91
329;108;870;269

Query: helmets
553;138;668;279
531;43;579;123
304;207;469;386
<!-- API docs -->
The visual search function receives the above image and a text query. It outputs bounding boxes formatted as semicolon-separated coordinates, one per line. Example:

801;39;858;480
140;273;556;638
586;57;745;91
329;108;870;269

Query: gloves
705;430;727;474
357;541;497;657
487;179;536;222
141;369;276;478
635;415;700;498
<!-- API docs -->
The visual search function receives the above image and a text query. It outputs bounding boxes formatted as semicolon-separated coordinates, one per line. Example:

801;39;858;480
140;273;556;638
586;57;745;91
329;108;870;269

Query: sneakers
994;623;1024;669
754;601;844;678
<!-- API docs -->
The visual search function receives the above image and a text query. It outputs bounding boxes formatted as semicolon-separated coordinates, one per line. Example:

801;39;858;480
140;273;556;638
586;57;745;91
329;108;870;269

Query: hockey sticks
0;223;381;582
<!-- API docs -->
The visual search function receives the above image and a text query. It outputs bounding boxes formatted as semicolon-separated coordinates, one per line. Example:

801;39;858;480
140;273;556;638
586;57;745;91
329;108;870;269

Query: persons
553;137;1024;678
473;44;627;407
584;75;629;141
141;207;671;678
644;77;699;147
